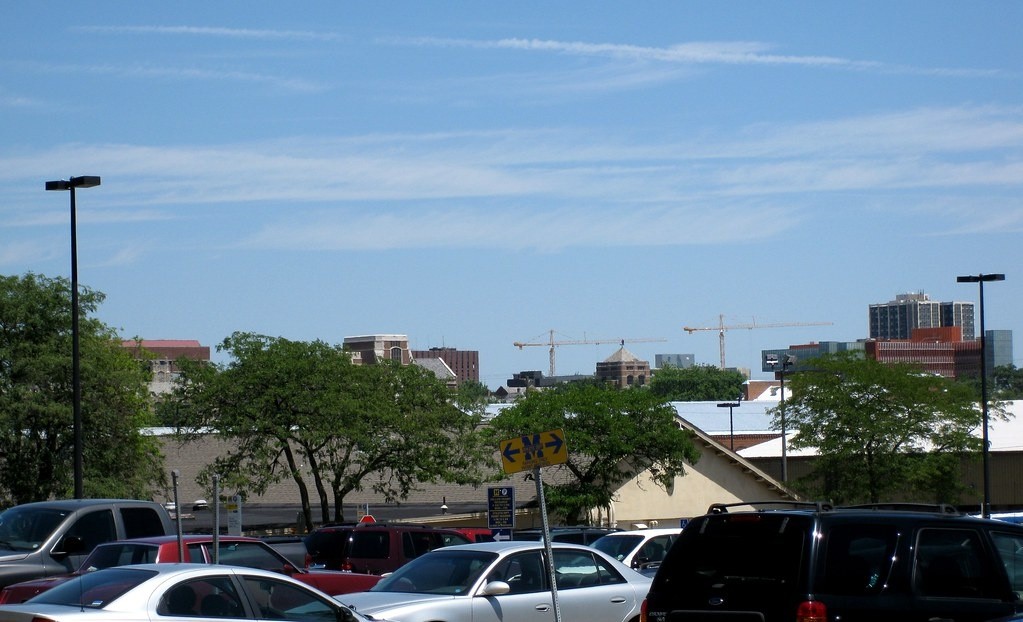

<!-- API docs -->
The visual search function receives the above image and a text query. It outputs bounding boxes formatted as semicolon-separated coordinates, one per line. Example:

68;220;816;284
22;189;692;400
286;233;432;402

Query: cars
0;562;398;621
0;534;388;604
282;540;652;622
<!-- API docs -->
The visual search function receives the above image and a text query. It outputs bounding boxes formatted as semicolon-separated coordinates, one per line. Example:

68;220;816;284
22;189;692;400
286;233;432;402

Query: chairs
165;585;247;619
712;534;995;606
467;557;610;595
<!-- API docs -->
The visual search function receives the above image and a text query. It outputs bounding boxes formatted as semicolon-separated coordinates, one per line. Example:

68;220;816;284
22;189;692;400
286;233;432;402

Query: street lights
716;403;740;450
44;176;102;498
957;274;1005;520
764;353;803;491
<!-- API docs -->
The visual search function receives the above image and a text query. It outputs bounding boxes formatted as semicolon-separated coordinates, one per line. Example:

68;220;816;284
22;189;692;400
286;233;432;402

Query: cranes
683;313;836;368
513;329;667;378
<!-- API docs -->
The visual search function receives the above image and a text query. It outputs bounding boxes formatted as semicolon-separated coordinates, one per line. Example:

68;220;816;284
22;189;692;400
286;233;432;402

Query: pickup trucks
0;496;313;594
298;521;479;574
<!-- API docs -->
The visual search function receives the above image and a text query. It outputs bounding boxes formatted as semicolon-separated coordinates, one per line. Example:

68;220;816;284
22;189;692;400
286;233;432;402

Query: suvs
466;525;686;581
634;497;1022;622
